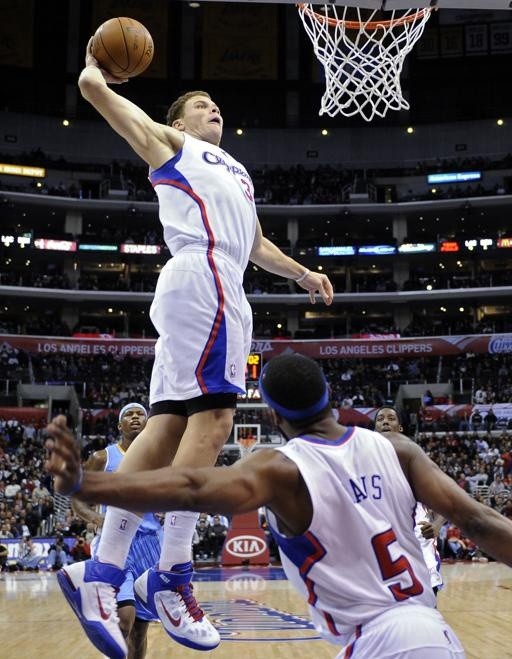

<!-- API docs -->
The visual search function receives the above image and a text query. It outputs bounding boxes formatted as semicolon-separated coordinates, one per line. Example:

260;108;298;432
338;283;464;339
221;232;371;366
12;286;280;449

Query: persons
56;35;333;659
72;402;161;659
43;352;512;659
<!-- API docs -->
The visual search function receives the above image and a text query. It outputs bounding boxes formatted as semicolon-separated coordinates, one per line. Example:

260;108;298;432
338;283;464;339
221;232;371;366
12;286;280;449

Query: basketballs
91;17;155;79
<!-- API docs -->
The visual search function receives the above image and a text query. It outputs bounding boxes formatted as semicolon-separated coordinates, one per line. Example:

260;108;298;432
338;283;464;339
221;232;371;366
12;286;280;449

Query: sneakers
56;554;129;659
133;561;221;651
